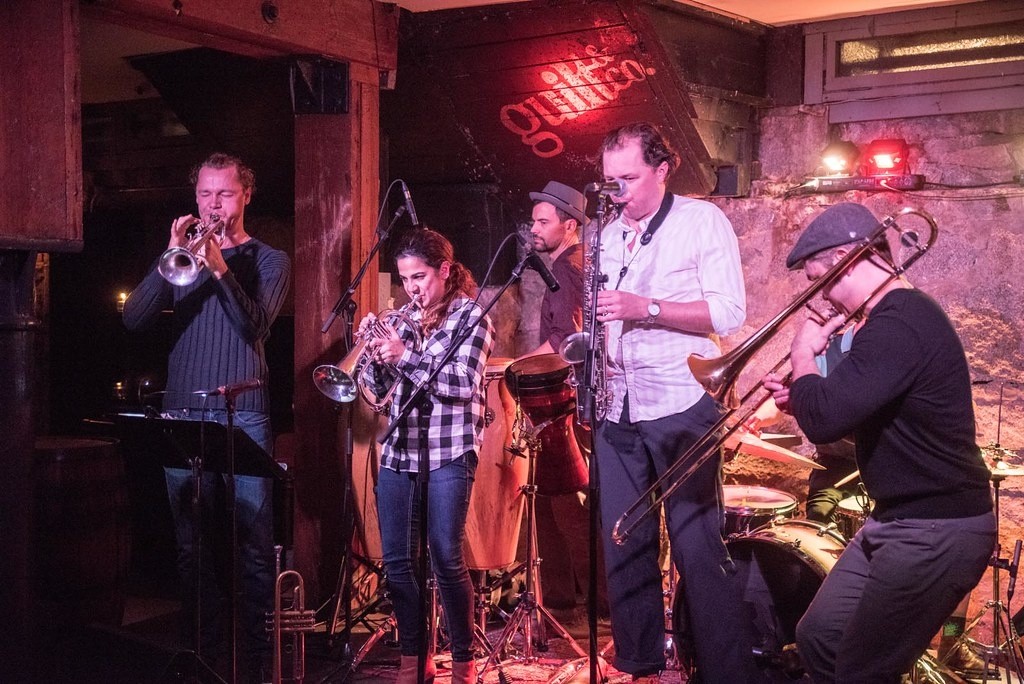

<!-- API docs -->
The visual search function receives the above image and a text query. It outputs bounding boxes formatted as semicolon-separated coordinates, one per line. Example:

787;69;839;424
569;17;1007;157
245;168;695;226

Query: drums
721;484;798;539
338;357;530;570
671;517;849;681
505;352;589;496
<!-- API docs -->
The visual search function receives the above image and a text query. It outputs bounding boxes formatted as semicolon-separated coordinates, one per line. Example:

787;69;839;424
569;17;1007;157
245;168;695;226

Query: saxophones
559;202;627;429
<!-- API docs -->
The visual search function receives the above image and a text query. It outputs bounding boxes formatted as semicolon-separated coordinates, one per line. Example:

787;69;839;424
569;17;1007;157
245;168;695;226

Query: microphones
401;182;420;225
208;378;265;396
1007;540;1023;600
518;236;561;293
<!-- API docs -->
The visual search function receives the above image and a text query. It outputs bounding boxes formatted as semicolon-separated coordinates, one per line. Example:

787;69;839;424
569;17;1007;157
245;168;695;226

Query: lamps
808;138;922;195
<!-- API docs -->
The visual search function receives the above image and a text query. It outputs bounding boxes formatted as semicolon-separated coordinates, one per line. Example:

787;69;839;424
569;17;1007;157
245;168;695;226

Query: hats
786;203;886;269
528;180;590;226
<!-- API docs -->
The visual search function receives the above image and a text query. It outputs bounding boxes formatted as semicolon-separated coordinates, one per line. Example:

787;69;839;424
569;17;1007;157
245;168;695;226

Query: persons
118;155;297;683
588;120;754;683
353;228;498;683
759;200;999;684
526;179;610;632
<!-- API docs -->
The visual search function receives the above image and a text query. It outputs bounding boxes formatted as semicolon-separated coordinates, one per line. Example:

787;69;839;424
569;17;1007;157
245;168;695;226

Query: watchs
645;298;662;325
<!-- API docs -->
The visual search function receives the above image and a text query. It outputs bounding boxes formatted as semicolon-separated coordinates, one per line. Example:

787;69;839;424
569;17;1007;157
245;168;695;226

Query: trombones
610;207;938;546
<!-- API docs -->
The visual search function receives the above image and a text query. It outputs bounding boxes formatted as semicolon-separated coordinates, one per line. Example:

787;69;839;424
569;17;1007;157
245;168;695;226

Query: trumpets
266;545;316;684
160;213;226;286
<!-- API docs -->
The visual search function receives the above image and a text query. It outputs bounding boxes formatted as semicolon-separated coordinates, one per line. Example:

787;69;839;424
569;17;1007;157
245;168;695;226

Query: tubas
313;293;423;412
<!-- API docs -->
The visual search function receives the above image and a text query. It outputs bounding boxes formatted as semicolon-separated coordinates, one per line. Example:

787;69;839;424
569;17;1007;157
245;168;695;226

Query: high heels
395;653;437;684
449;658;478;684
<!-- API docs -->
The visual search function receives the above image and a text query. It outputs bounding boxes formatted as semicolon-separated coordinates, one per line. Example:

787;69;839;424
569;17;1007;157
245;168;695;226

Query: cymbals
984;461;1024;479
